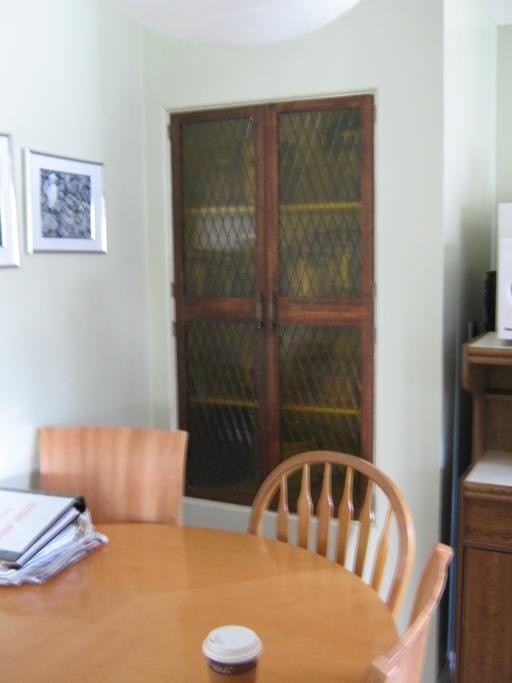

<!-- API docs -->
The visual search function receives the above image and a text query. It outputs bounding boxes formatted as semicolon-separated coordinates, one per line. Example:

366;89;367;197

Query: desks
1;519;400;682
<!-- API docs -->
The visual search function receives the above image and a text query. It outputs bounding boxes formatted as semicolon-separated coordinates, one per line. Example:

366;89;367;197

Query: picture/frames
21;145;107;252
0;132;22;266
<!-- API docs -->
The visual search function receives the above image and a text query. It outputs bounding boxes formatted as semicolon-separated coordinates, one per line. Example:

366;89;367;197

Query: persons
43;173;58;213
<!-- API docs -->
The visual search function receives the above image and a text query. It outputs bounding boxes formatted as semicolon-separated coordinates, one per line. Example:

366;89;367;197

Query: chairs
361;541;456;681
39;426;188;525
245;449;414;617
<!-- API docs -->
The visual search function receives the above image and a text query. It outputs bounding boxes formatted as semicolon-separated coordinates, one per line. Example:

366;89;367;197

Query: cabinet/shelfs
168;93;375;520
461;327;512;680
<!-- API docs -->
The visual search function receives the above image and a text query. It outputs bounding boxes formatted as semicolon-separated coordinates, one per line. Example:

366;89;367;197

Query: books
0;486;107;586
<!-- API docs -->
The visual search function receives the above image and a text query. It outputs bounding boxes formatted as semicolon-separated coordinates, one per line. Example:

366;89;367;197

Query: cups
205;625;262;683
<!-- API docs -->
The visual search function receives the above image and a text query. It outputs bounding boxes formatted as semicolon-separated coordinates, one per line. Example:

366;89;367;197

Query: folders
0;487;86;568
